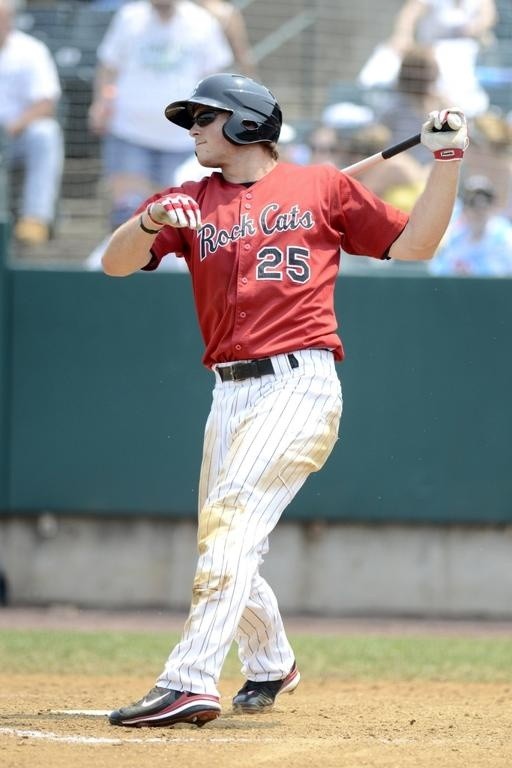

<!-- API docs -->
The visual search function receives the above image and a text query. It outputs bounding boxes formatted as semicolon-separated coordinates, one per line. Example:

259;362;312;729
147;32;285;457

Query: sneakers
110;686;221;728
232;660;300;714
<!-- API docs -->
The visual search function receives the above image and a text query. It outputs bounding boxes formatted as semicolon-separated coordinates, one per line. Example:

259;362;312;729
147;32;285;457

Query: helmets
165;73;281;145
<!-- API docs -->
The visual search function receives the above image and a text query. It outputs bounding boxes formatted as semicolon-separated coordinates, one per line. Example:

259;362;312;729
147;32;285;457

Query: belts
216;354;298;381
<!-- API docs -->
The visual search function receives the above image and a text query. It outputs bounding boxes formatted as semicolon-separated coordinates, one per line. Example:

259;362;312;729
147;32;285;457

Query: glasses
188;110;223;129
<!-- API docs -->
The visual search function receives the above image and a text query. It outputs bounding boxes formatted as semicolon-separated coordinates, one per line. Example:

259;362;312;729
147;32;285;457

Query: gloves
147;193;201;230
420;109;467;161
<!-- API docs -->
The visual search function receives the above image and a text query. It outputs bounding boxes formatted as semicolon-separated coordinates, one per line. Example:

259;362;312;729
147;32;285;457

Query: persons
0;2;65;242
315;0;512;278
100;74;469;728
88;1;253;229
